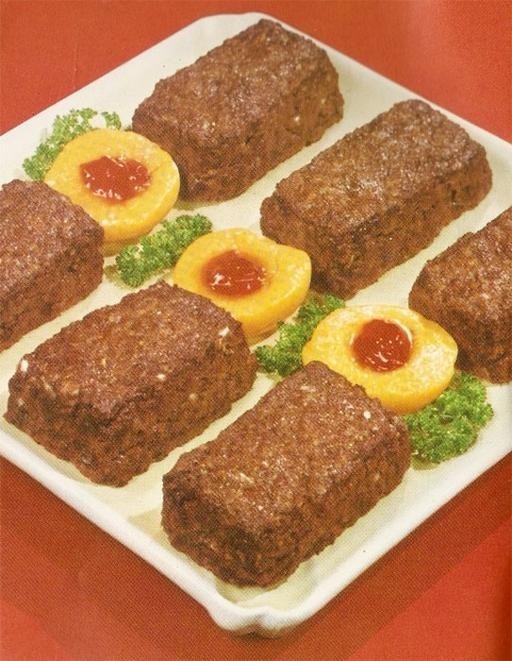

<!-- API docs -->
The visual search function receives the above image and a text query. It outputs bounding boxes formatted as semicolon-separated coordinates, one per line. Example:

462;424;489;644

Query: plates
2;9;511;643
